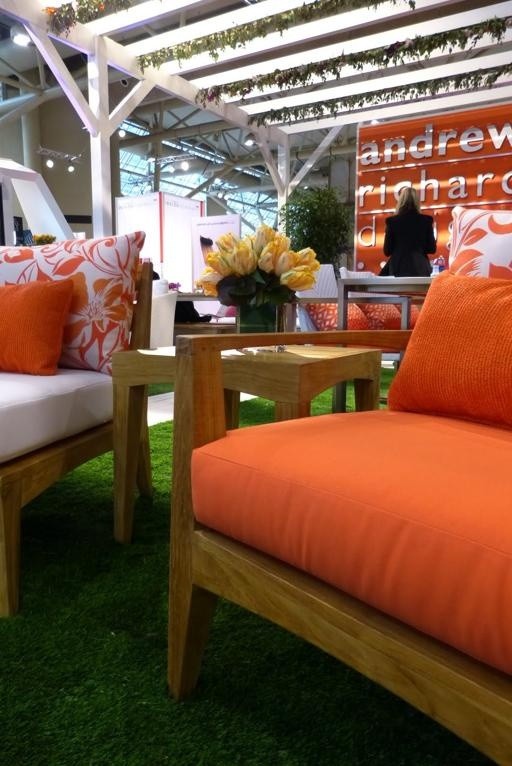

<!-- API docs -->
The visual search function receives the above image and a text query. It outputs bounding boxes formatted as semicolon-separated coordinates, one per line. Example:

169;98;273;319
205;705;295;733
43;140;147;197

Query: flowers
194;224;322;309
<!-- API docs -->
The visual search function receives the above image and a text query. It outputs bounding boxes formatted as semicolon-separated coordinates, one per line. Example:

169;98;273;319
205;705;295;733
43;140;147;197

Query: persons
384;186;436;277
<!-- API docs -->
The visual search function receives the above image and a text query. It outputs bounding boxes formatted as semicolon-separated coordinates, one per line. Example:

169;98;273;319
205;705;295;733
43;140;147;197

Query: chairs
155;204;511;762
295;258;422;362
0;227;156;618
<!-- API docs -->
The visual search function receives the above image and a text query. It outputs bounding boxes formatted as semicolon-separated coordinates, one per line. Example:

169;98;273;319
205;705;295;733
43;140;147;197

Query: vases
236;304;287;354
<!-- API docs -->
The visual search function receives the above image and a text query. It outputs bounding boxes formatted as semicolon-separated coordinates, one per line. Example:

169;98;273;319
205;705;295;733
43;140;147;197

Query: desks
107;344;383;550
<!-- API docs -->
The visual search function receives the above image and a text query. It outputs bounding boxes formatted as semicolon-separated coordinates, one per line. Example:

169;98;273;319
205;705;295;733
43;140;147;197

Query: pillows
384;207;510;428
0;229;150;382
